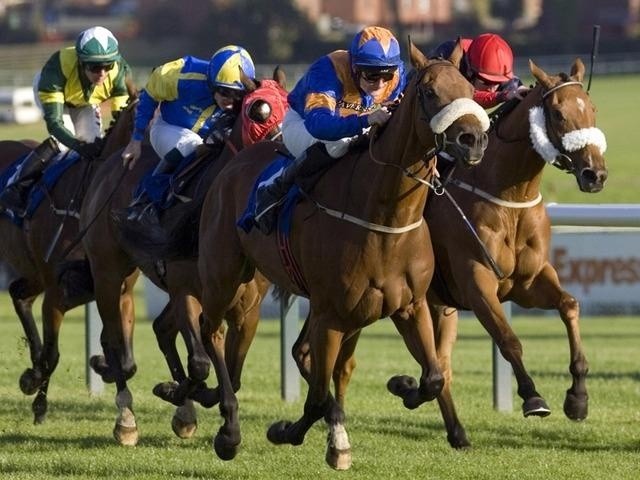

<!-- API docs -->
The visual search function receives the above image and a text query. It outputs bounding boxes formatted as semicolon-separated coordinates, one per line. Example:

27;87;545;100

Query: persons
121;45;255;222
0;26;138;213
406;33;532;109
255;27;406;235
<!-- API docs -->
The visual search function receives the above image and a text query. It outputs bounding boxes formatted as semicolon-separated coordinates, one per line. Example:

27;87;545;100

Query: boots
254;141;335;234
136;146;187;197
1;136;59;207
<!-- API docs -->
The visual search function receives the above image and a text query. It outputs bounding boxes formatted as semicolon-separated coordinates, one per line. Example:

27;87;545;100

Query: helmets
351;26;403;66
469;33;513;82
208;45;255;90
75;26;119;62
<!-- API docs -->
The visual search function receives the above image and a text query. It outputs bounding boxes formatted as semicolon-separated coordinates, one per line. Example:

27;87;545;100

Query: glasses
216;85;245;98
474;74;508;87
87;63;116;73
357;69;396;84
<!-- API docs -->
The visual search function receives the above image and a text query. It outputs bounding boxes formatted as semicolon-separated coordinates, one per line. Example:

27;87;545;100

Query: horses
2;91;140;426
77;64;288;448
331;58;610;450
197;35;490;471
